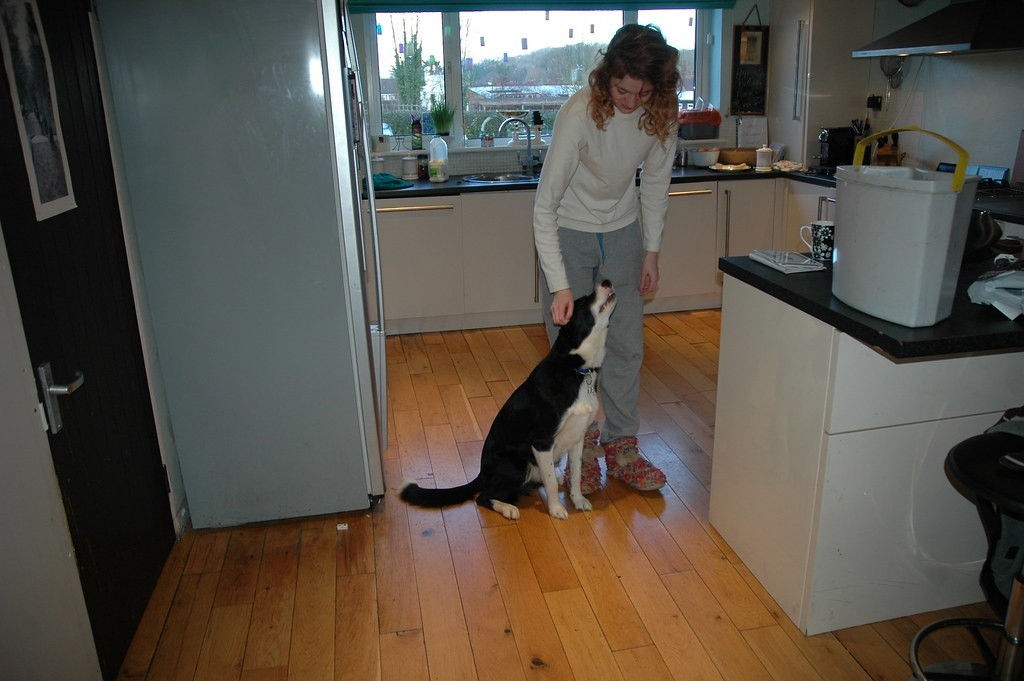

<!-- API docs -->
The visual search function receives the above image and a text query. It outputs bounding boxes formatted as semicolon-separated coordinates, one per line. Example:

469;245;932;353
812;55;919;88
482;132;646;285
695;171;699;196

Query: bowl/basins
689;149;721;167
720;148;759;167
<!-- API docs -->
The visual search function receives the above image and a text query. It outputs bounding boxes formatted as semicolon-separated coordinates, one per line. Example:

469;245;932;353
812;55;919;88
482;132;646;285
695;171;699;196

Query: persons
532;25;680;494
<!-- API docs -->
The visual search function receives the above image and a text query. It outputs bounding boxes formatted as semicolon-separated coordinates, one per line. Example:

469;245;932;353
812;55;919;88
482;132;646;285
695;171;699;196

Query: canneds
480;133;495;147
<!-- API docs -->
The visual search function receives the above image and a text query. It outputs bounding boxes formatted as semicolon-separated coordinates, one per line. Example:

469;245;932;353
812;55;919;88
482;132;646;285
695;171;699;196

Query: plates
708;165;751;171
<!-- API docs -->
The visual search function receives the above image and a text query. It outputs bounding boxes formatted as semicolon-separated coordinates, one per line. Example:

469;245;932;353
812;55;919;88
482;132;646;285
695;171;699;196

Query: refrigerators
86;0;390;530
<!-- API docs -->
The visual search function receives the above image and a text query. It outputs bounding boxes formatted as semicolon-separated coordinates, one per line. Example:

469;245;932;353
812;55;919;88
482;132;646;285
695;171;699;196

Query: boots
602;436;666;489
564;430;601;494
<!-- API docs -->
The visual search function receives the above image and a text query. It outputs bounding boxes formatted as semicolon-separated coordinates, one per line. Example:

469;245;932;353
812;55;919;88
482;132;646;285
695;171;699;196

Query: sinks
462;173;539;183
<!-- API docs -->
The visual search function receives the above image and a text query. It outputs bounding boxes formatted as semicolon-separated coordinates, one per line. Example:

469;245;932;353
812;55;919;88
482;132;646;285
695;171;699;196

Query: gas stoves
936;161;1023;198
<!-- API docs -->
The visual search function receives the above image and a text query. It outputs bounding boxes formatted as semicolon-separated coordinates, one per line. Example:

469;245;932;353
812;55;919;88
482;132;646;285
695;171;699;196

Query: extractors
852;0;1024;59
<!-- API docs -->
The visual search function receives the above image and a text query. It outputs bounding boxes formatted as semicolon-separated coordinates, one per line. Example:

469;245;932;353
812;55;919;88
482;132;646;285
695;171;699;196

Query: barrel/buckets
829;126;983;329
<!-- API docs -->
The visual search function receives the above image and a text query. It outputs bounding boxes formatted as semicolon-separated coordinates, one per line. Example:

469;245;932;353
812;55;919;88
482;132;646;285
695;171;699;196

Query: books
749;249;823;273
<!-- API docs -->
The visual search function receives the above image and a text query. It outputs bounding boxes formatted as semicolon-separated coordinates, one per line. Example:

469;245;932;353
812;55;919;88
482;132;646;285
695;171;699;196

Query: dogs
399;280;619;521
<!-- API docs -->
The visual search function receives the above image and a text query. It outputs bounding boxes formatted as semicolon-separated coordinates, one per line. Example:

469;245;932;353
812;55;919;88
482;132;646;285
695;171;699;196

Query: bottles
679;147;688;166
481;132;495;147
428;135;450;182
417;154;428;179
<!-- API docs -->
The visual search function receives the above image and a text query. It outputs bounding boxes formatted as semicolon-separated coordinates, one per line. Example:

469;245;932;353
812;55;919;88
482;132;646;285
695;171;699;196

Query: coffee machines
807;124;871;175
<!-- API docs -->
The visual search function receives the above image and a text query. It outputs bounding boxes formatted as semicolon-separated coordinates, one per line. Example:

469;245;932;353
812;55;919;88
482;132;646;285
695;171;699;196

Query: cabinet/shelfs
361;178;1024;335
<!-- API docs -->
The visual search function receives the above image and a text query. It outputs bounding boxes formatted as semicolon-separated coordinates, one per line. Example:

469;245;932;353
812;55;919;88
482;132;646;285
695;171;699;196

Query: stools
910;432;1024;681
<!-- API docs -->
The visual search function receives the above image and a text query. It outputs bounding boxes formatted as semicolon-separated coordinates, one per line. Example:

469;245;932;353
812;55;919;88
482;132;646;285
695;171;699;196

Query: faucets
498;117;533;174
480;115;503;137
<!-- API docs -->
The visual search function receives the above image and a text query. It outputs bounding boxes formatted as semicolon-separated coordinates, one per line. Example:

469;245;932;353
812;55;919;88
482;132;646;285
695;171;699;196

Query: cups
755;144;772;171
800;220;835;261
402;153;418;179
